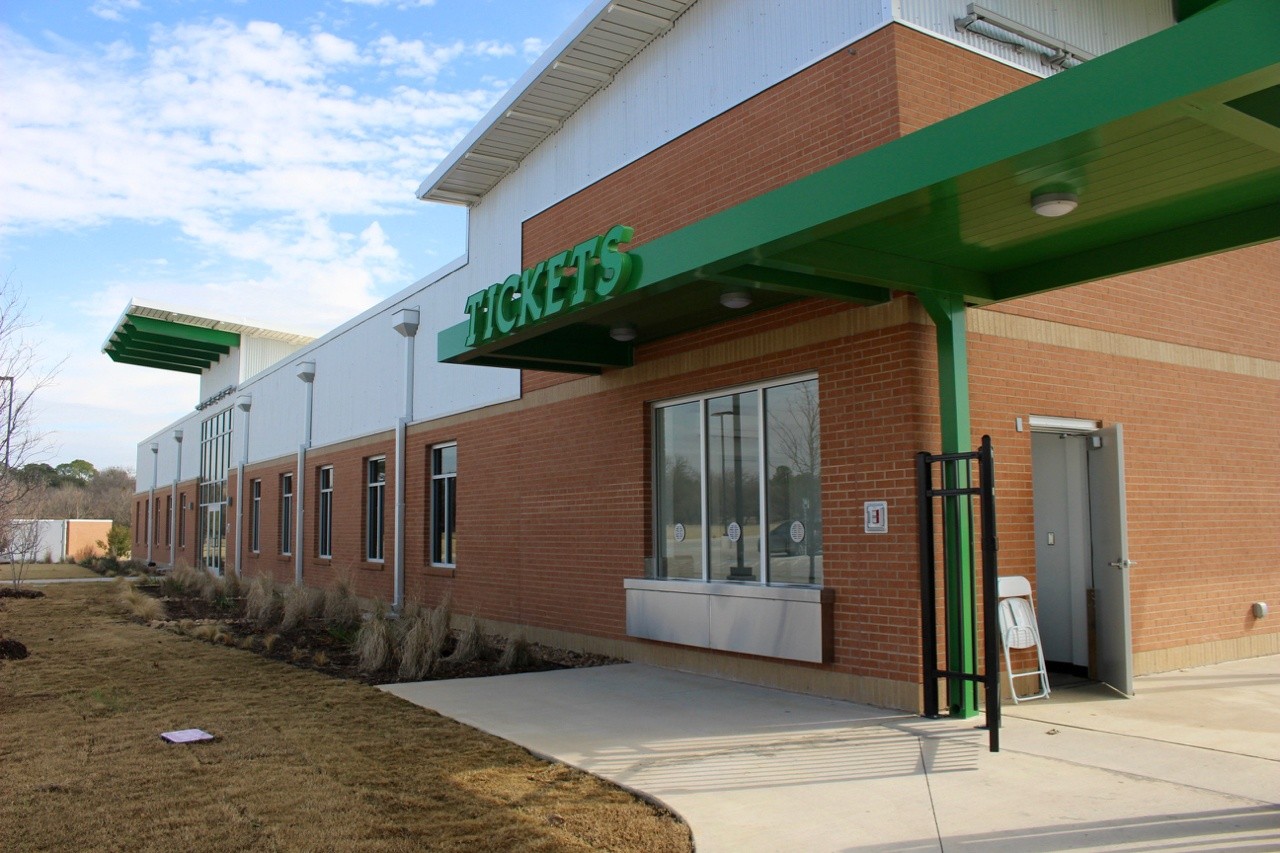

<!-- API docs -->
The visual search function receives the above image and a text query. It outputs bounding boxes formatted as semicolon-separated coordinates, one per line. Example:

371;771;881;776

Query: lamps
1031;193;1077;217
719;292;752;309
609;329;635;341
